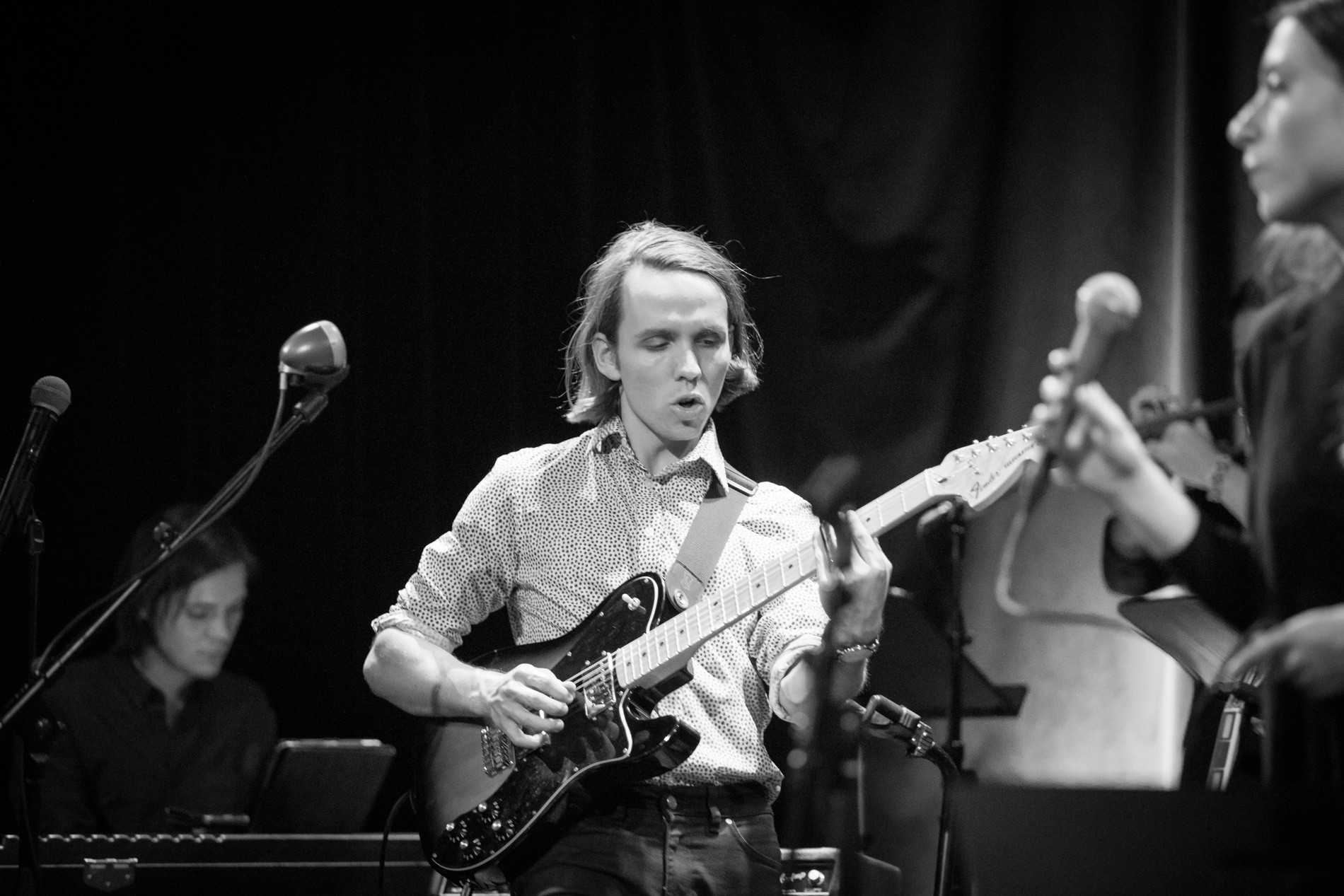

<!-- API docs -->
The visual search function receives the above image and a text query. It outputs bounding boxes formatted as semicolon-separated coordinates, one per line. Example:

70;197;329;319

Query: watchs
822;622;880;667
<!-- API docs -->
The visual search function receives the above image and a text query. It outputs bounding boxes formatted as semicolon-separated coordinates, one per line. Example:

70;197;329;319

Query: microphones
0;375;70;516
1020;272;1142;513
279;319;348;379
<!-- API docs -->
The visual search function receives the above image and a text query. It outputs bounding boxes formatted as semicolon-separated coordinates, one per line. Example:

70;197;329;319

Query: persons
1029;2;1344;896
362;221;894;896
6;485;289;837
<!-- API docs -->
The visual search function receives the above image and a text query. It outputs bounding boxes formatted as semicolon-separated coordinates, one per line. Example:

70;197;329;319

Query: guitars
413;421;1056;877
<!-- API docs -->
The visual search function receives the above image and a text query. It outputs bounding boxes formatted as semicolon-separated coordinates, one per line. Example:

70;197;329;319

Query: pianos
0;821;445;894
